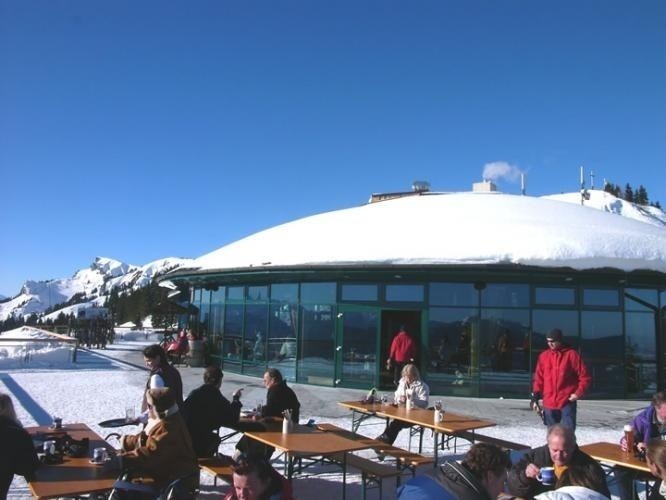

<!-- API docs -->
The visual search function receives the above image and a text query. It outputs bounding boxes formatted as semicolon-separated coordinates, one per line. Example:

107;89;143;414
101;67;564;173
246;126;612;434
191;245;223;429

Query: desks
22;420;156;499
576;441;666;499
338;398;500;467
217;408;371;500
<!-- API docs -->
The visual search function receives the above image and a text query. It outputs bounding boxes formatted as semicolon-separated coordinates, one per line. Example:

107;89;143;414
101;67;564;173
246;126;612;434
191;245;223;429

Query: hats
546;327;562;342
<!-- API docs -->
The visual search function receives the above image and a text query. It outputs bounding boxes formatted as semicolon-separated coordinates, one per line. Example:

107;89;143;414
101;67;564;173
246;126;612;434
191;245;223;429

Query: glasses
546;339;558;343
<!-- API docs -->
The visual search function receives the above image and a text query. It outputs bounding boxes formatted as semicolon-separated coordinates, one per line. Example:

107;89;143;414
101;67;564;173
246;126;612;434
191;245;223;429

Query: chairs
183;335;208;369
169;339;184;361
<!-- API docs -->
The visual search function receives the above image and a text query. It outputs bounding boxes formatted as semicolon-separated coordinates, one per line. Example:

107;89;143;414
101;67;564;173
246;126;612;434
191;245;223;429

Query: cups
52;417;63;428
93;448;108;462
282;419;294;433
434;410;444;423
125;404;136;422
624;431;634;453
42;441;55;455
405;399;415;411
255;399;263;415
536;467;554;487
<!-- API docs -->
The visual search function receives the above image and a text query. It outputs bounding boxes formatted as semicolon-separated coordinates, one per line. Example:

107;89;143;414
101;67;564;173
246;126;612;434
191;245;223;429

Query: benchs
416;423;532;455
319;452;402;499
197;455;234;478
312;423;437;489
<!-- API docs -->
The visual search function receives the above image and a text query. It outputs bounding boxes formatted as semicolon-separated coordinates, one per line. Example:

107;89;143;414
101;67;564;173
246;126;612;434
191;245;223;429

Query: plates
89;458;112;466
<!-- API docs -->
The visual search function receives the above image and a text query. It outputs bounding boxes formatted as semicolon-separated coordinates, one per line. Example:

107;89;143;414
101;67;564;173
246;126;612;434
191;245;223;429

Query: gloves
534;406;546;422
529;390;541;411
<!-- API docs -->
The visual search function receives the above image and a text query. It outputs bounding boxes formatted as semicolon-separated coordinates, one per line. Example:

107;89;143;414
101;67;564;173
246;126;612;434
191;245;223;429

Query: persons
645;440;666;500
371;363;429;461
529;329;592;434
227;327;297;364
236;368;301;463
530;462;612;500
394;443;511;500
432;330;530;373
104;388;195;500
506;424;610;500
132;345;182;448
0;394;39;500
620;391;666;500
386;325;416;386
223;456;293;500
183;367;244;459
